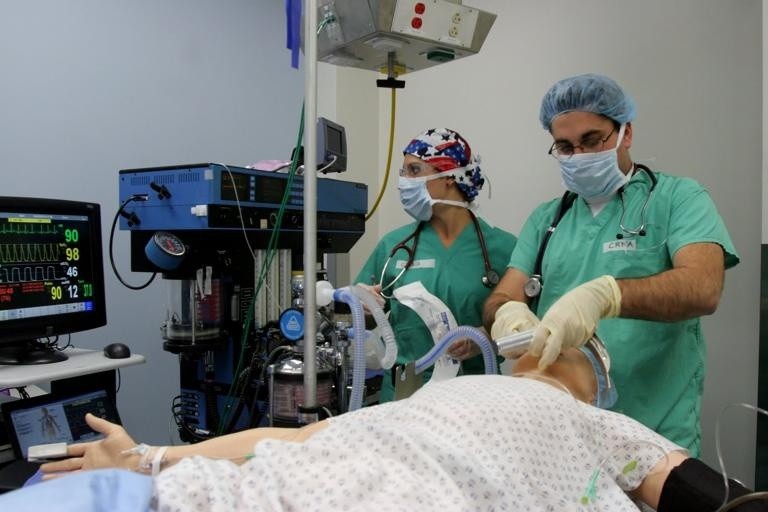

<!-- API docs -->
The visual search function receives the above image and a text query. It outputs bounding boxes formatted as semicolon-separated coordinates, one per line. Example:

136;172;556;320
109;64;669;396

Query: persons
348;127;525;399
481;72;741;463
1;326;768;510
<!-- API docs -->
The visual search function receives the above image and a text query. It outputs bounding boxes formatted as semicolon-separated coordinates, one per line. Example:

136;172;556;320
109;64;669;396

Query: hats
538;72;637;131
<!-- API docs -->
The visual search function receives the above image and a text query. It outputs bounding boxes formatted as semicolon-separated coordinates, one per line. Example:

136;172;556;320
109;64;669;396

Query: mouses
104;343;130;358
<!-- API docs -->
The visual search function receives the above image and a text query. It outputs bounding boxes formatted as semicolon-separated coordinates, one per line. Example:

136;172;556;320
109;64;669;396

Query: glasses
548;129;617;165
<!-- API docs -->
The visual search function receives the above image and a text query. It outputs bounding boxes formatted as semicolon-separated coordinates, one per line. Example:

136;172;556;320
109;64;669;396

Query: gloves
491;298;541;361
527;274;622;371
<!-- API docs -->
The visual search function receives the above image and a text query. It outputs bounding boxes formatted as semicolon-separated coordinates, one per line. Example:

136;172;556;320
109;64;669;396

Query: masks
556;149;627;198
396;174;436;224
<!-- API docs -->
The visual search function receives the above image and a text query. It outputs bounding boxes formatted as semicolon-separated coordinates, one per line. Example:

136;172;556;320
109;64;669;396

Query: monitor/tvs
9;389;123;461
0;196;107;365
